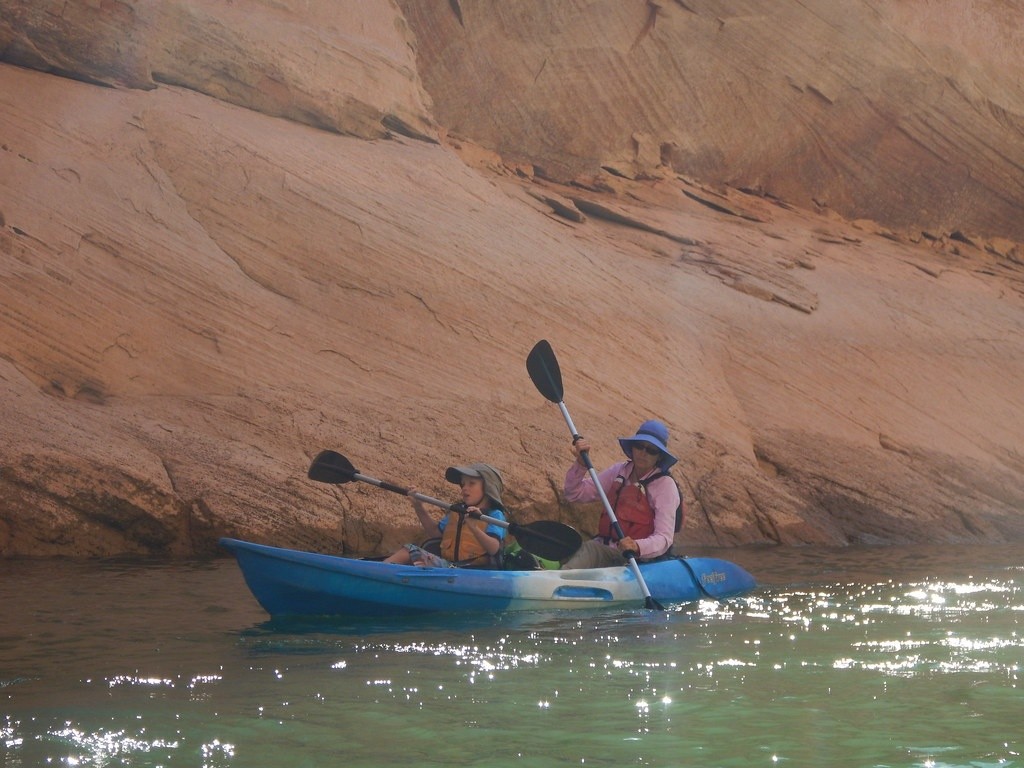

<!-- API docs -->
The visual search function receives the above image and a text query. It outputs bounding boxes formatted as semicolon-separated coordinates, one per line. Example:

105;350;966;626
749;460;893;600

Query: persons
385;463;509;570
559;419;682;570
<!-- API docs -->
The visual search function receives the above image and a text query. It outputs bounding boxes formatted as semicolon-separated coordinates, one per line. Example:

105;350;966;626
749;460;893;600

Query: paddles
307;449;583;561
525;339;668;611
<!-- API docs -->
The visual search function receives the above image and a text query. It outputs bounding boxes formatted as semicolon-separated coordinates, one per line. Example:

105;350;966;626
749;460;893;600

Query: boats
218;535;758;632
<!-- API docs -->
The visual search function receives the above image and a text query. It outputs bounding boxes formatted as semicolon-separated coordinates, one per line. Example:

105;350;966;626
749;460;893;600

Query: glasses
633;442;659;455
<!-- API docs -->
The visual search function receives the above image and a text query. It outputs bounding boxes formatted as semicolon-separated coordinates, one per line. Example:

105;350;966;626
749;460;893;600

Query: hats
446;460;505;506
619;421;678;468
